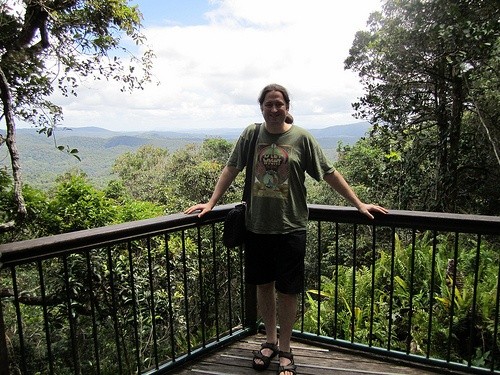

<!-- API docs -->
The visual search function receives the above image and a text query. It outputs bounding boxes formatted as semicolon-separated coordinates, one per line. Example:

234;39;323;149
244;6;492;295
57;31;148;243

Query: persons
184;84;389;375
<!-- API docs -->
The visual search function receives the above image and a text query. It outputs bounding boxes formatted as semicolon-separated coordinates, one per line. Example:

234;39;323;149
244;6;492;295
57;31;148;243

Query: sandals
276;348;298;375
252;341;278;372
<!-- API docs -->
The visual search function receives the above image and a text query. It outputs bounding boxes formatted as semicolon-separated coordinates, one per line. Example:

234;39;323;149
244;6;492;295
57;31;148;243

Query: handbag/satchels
223;204;246;250
